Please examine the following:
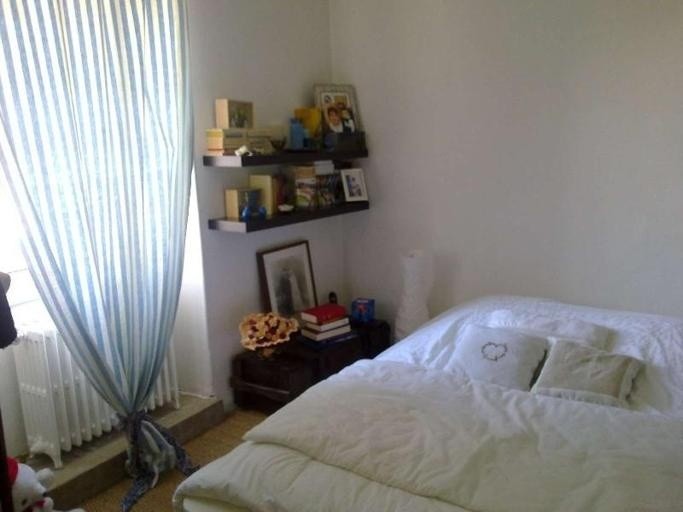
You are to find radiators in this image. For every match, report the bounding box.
[10,320,181,470]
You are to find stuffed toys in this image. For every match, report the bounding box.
[5,455,85,512]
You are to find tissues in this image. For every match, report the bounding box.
[352,297,375,322]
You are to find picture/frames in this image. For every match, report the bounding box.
[339,168,369,203]
[255,239,318,330]
[313,84,363,132]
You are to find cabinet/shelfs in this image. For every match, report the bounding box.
[229,315,391,412]
[202,147,369,233]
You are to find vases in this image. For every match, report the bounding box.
[247,349,280,367]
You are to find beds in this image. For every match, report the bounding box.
[171,290,683,512]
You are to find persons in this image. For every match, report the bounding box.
[349,176,362,197]
[277,266,291,315]
[323,102,343,133]
[340,109,355,136]
[288,269,305,313]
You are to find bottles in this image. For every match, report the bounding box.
[289,117,304,150]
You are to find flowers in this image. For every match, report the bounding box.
[238,311,300,352]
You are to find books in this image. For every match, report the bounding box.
[248,173,286,219]
[296,304,359,352]
[205,128,273,158]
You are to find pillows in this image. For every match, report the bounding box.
[534,337,642,408]
[441,323,548,391]
[490,308,612,349]
